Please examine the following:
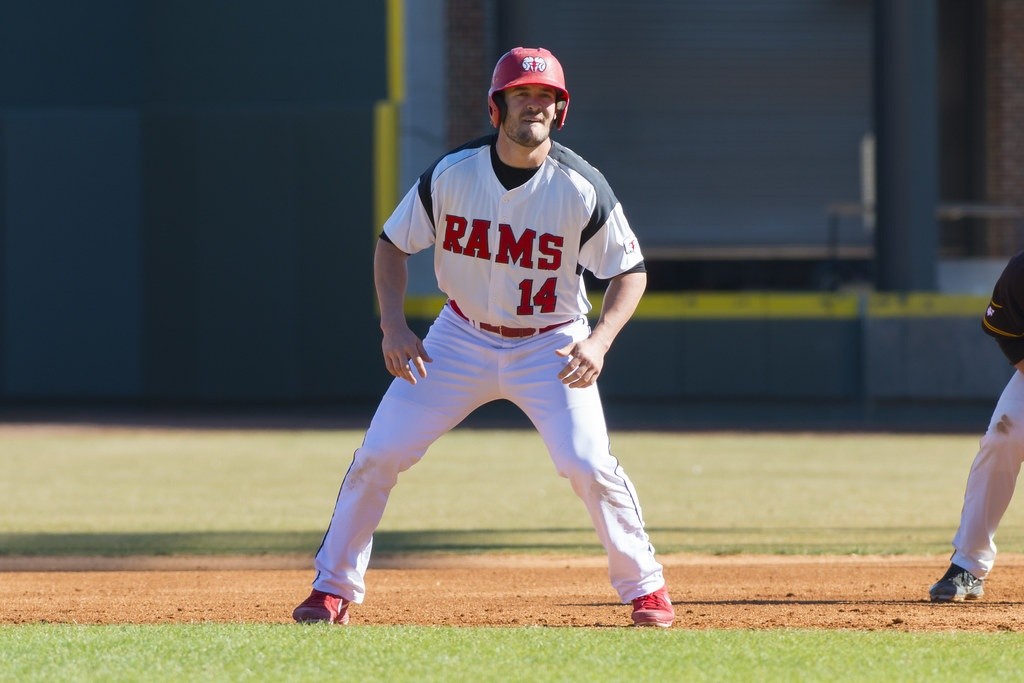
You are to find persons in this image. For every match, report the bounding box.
[929,252,1024,601]
[292,47,676,629]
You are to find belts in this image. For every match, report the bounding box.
[450,300,574,337]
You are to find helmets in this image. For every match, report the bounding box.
[487,46,570,131]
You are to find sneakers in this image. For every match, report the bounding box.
[631,584,675,627]
[293,587,349,625]
[929,563,985,603]
